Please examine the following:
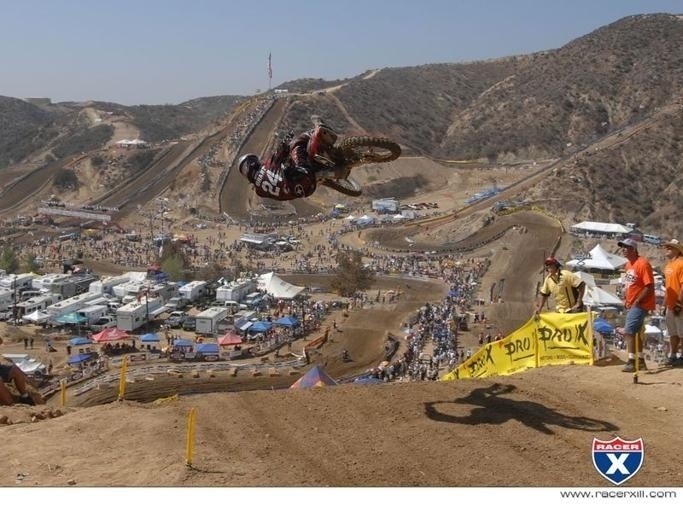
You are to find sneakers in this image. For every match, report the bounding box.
[311,115,324,126]
[21,396,36,406]
[662,356,683,367]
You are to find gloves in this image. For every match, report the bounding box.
[672,300,681,316]
[663,308,666,316]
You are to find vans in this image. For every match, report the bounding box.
[643,233,662,248]
[224,292,262,311]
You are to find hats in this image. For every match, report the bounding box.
[617,239,637,248]
[660,239,683,256]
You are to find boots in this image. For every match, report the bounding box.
[622,357,647,372]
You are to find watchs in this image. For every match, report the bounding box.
[635,299,640,304]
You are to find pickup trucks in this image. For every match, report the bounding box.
[90,316,117,332]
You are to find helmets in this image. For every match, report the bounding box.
[238,153,259,176]
[545,256,558,265]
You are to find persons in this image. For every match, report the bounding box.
[238,115,332,202]
[617,238,656,372]
[535,256,585,313]
[0,209,505,406]
[661,238,683,368]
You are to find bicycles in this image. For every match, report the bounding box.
[277,115,402,197]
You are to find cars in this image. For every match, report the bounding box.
[164,296,196,330]
[289,236,300,245]
[410,202,440,210]
